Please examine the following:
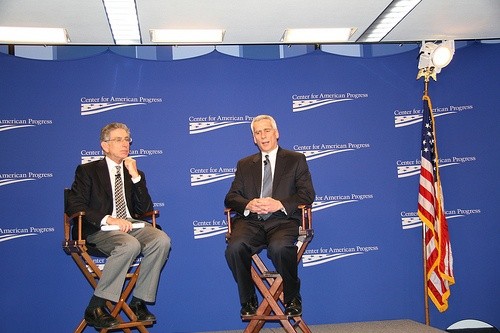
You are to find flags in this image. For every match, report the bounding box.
[417,95,455,312]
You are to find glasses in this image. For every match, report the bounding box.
[105,137,133,144]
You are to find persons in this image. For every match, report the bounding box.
[226,114,316,317]
[66,122,170,327]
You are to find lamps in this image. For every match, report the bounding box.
[419,42,455,71]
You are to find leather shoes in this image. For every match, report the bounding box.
[284,295,302,315]
[128,296,156,322]
[240,294,258,316]
[84,305,120,326]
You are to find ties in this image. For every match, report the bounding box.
[115,166,128,221]
[259,155,273,221]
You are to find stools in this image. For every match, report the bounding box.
[63,188,160,333]
[225,203,313,333]
